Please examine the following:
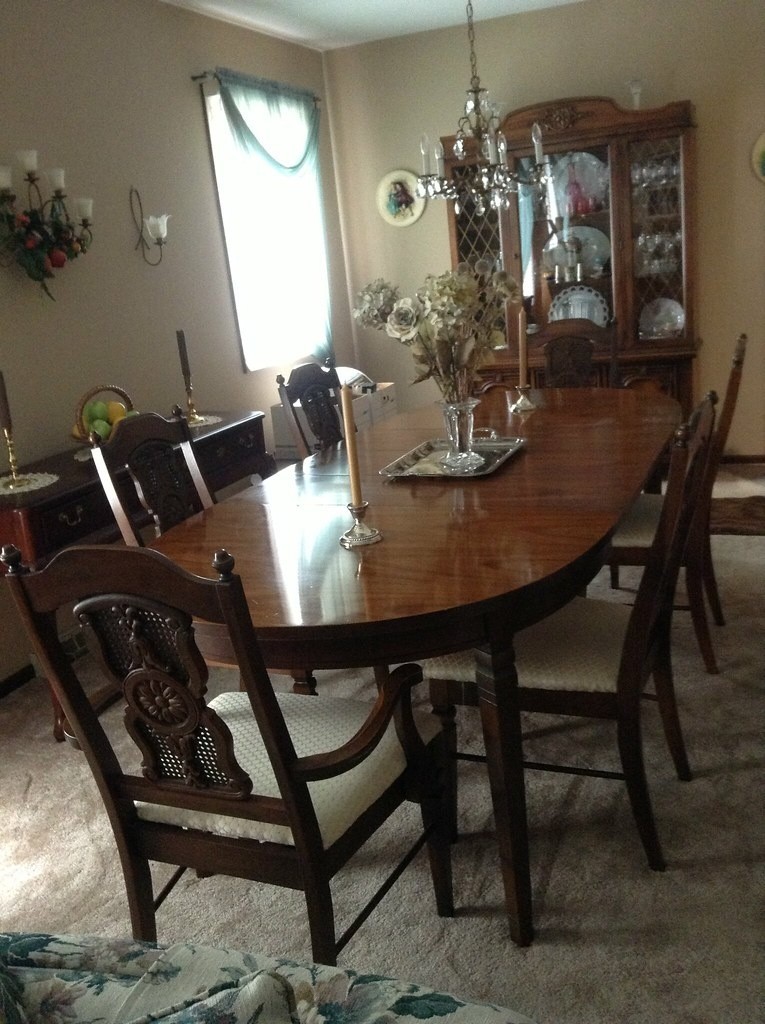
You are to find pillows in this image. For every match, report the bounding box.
[129,969,301,1024]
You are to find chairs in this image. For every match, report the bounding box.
[526,316,619,389]
[387,332,748,949]
[0,543,457,968]
[87,403,391,704]
[279,356,362,461]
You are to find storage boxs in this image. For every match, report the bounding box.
[271,381,397,460]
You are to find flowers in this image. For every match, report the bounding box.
[348,264,520,400]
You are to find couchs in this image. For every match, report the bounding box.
[0,931,538,1024]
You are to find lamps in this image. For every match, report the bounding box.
[0,146,93,269]
[415,0,551,218]
[130,184,172,268]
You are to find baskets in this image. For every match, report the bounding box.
[71,384,134,447]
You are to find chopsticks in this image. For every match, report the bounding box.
[540,226,611,279]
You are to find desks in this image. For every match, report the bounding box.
[0,408,276,742]
[105,384,682,942]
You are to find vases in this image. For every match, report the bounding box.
[437,395,486,473]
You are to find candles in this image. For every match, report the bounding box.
[0,370,12,428]
[340,380,363,507]
[176,330,190,377]
[518,306,527,388]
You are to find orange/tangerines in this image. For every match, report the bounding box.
[108,402,127,428]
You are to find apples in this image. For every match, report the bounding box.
[72,401,111,440]
[126,411,140,417]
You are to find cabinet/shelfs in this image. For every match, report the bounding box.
[438,93,704,483]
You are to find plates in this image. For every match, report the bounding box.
[548,285,610,322]
[638,298,684,340]
[546,152,610,216]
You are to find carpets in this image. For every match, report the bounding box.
[710,495,765,536]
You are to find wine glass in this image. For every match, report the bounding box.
[629,160,682,274]
